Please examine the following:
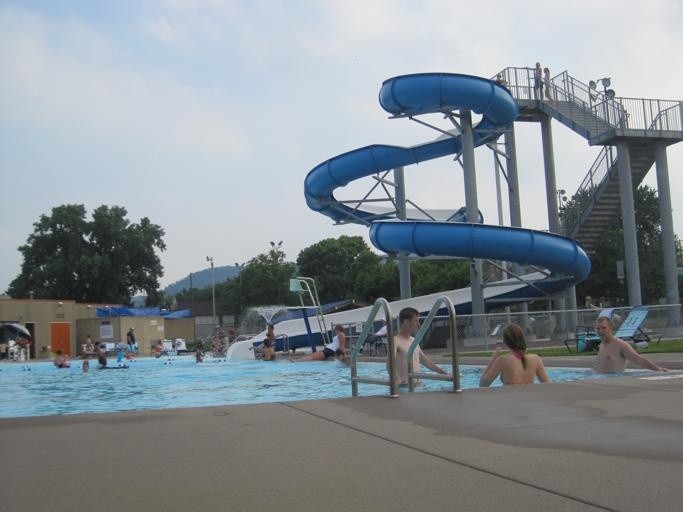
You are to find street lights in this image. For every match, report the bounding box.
[207,255,219,325]
[271,240,282,301]
[233,262,245,312]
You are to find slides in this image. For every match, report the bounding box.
[254,72,591,361]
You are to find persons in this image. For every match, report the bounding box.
[288,324,346,362]
[533,63,543,100]
[385,306,452,387]
[593,316,669,372]
[496,73,502,84]
[544,67,553,100]
[0,325,277,371]
[357,317,388,356]
[479,322,550,387]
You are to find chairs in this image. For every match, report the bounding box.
[362,319,387,357]
[564,306,650,355]
[162,336,191,356]
